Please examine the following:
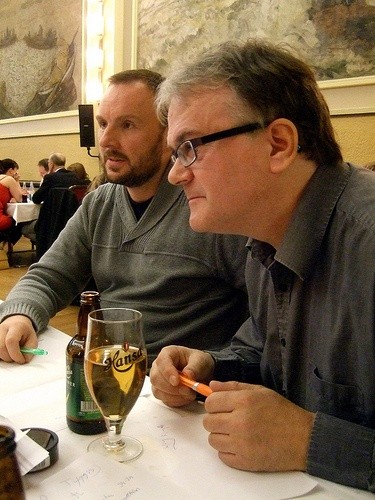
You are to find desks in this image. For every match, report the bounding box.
[5,197,44,266]
[0,299,375,500]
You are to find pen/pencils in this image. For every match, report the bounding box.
[179,374,213,398]
[20,347,48,355]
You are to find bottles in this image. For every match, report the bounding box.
[0,424,26,500]
[29,182,35,201]
[65,290,113,436]
[22,182,28,203]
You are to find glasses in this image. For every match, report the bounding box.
[170,119,303,167]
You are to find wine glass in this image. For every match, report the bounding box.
[83,308,148,463]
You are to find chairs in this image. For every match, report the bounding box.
[34,185,88,259]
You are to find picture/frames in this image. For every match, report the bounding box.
[0,0,86,125]
[131,0,375,89]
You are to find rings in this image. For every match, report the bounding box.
[16,172,17,174]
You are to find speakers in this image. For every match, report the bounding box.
[79,104,95,147]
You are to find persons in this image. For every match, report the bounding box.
[21,152,91,253]
[150,37,375,494]
[0,158,23,249]
[0,68,249,376]
